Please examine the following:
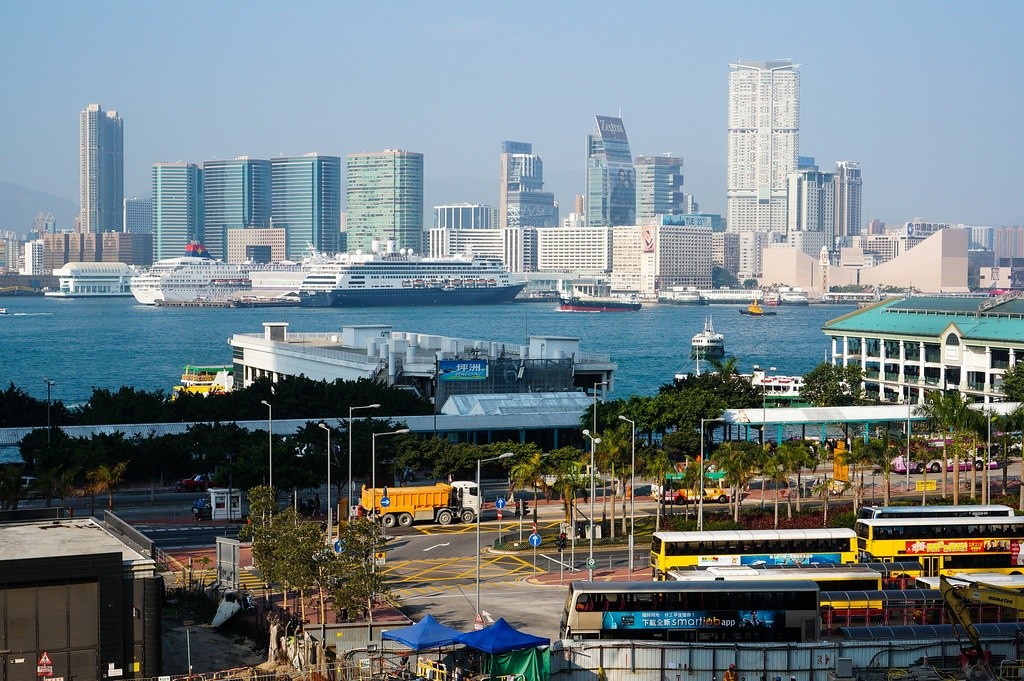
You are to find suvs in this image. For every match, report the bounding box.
[181,472,222,492]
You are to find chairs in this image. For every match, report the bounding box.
[582,594,691,611]
[873,527,1015,536]
[667,538,851,555]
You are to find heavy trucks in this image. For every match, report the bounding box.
[361,476,486,529]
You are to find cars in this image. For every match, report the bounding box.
[16,476,44,500]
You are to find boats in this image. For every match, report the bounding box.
[411,274,498,291]
[739,285,782,316]
[673,344,850,404]
[689,313,726,360]
[699,294,710,305]
[552,283,643,313]
[169,357,234,403]
[42,237,530,308]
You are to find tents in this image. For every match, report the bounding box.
[380,613,550,676]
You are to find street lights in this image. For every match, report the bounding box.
[318,423,332,591]
[476,451,515,623]
[594,381,607,501]
[372,427,411,603]
[619,415,635,570]
[348,403,381,537]
[698,417,726,532]
[42,376,55,450]
[581,428,601,582]
[260,399,272,531]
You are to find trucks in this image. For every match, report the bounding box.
[533,464,602,489]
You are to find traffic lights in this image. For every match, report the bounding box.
[514,501,530,517]
[555,533,567,551]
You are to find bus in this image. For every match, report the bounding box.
[650,472,744,504]
[557,503,1024,643]
[887,431,1024,475]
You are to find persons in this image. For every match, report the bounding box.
[674,463,686,473]
[174,480,182,493]
[765,440,774,453]
[608,168,636,227]
[749,611,761,626]
[984,540,1010,552]
[358,494,362,505]
[643,229,654,252]
[312,493,324,517]
[817,433,852,461]
[790,435,801,441]
[722,663,739,681]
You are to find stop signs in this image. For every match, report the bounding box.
[532,522,537,534]
[497,510,503,521]
